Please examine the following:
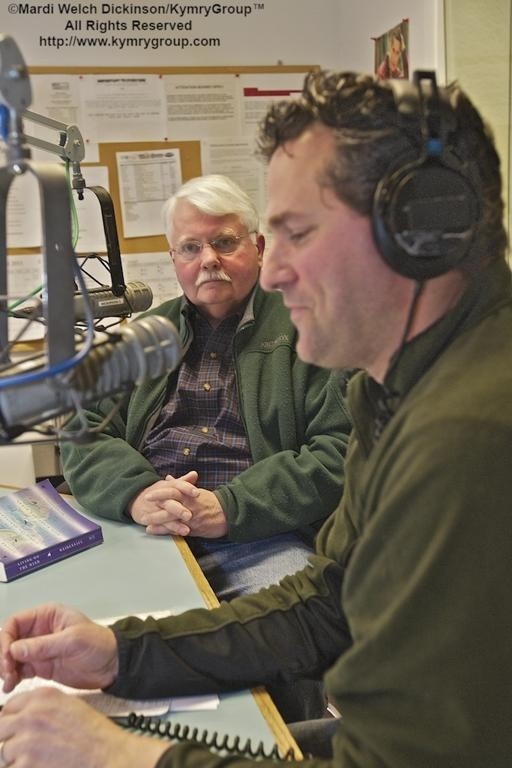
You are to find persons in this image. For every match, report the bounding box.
[59,175,352,600]
[0,65,511,768]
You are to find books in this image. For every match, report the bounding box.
[0,478,103,582]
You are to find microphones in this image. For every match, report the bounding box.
[9,283,152,317]
[2,316,183,442]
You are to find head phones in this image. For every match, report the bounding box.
[369,67,480,285]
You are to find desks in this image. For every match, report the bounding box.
[0,456,316,766]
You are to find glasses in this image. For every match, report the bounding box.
[169,230,259,260]
